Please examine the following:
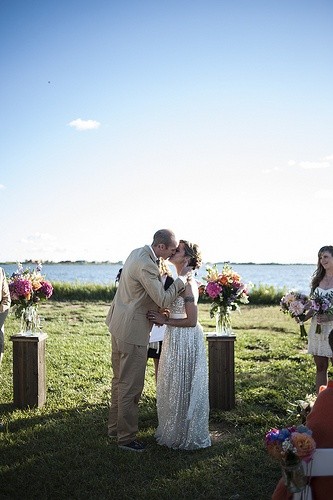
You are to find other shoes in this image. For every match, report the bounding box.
[121,438,148,453]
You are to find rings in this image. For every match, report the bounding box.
[153,317,155,319]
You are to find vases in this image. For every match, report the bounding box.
[216,308,232,336]
[283,464,307,493]
[20,305,40,335]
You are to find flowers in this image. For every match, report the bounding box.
[6,260,54,305]
[263,424,317,463]
[199,261,249,318]
[280,288,333,338]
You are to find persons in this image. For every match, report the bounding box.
[146,240,211,450]
[147,260,174,384]
[295,245,333,394]
[104,230,192,452]
[0,267,10,366]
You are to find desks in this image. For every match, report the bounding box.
[10,333,49,408]
[206,334,237,413]
